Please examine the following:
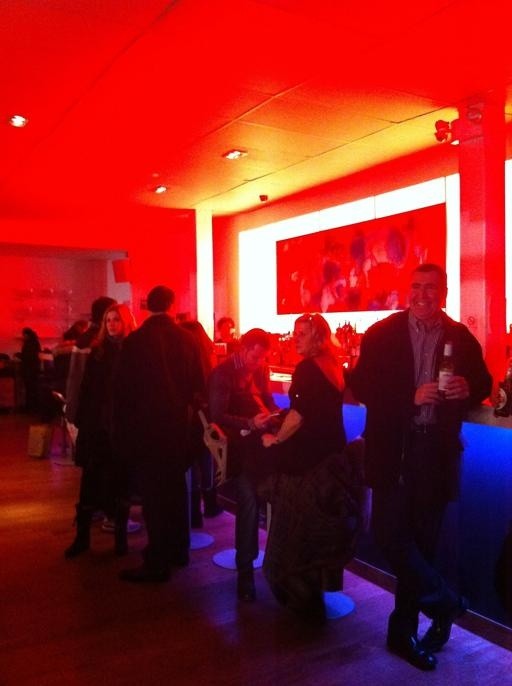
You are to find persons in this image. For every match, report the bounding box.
[18,319,84,425]
[348,265,491,670]
[205,313,346,641]
[318,230,408,310]
[64,284,238,581]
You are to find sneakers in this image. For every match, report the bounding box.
[235,556,256,593]
[120,566,170,582]
[115,532,127,555]
[101,518,141,533]
[141,546,187,567]
[64,538,89,556]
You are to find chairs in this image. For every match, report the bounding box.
[50,389,67,407]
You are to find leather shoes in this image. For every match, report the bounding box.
[386,629,437,669]
[421,597,468,651]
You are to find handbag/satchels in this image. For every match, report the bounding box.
[195,421,229,492]
[28,427,51,458]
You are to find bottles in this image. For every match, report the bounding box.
[494,363,512,418]
[437,339,455,398]
